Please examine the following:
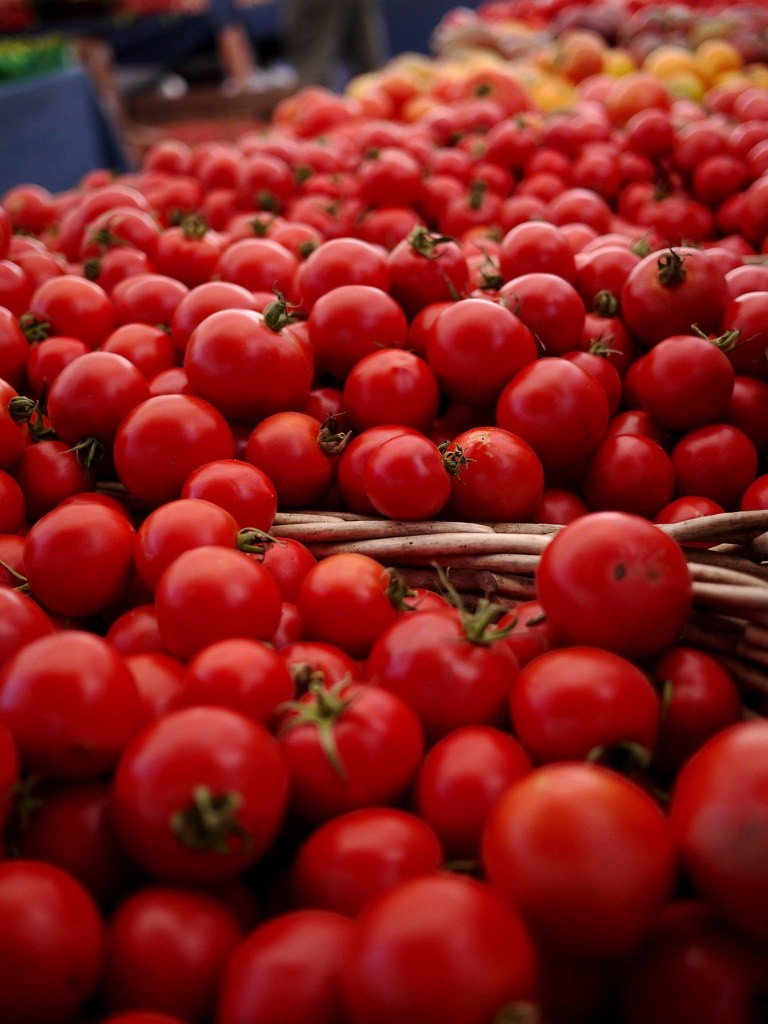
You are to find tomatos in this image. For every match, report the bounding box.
[0,0,768,1024]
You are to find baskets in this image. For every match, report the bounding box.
[25,469,768,532]
[0,534,768,721]
